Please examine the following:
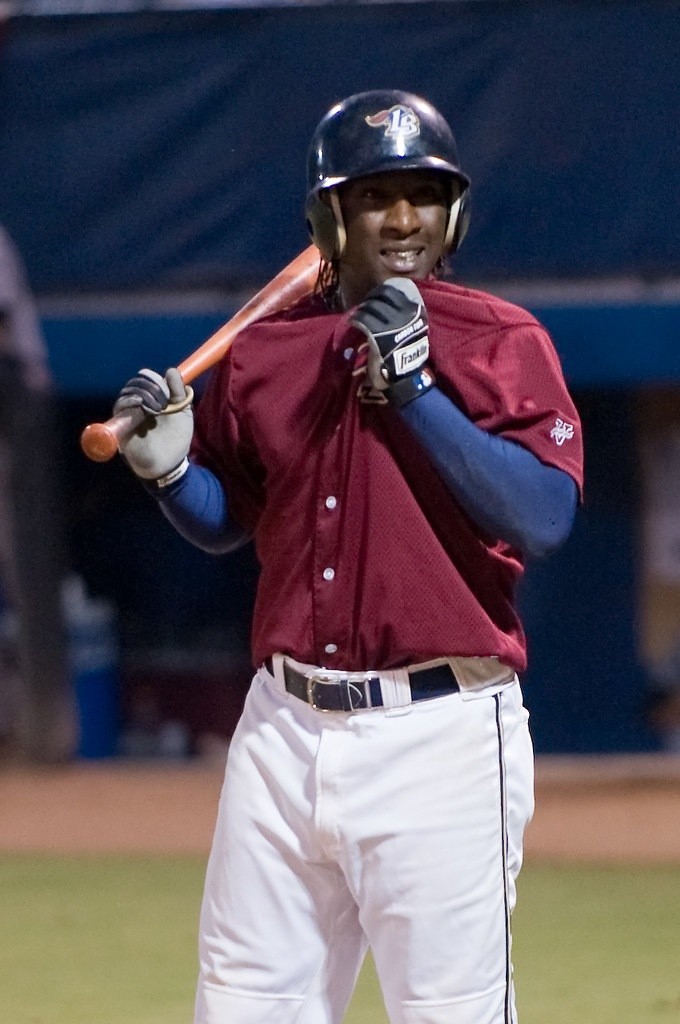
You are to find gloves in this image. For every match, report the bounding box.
[114,367,196,491]
[348,276,438,412]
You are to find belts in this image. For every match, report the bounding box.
[263,657,460,713]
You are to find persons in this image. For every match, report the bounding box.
[0,224,83,763]
[114,90,581,1023]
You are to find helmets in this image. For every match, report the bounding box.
[300,88,472,263]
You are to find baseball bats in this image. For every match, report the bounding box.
[78,243,338,463]
